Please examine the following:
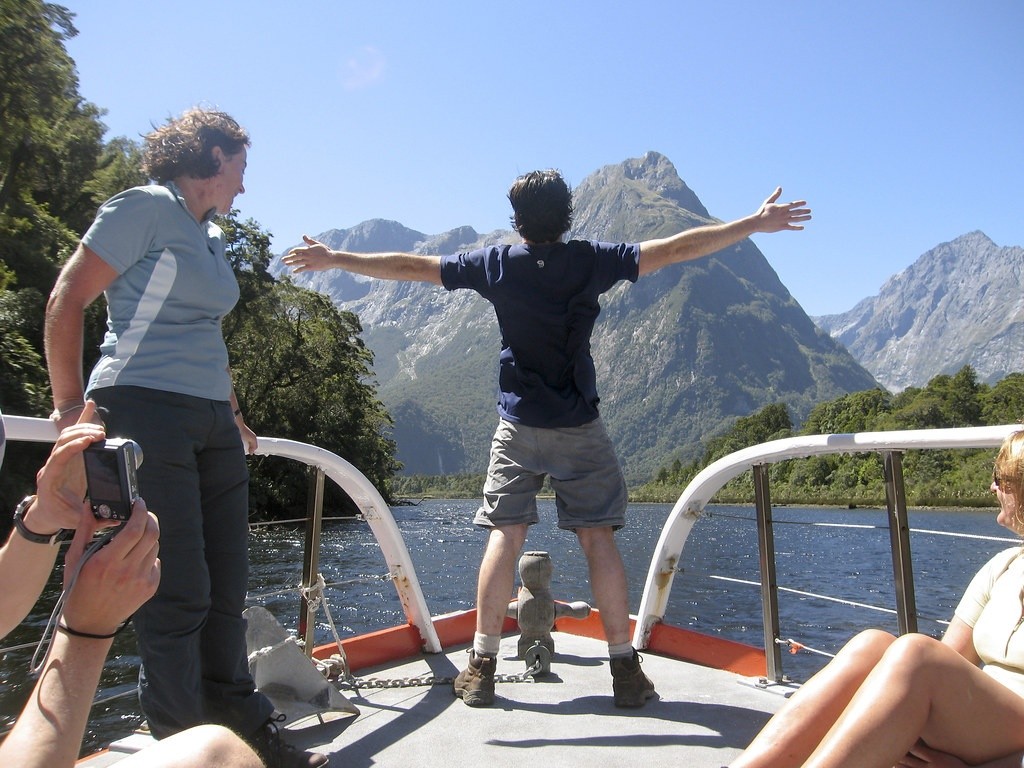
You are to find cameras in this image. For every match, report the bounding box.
[83,439,145,522]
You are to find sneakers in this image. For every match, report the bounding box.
[610,646,654,707]
[247,714,330,768]
[453,646,497,707]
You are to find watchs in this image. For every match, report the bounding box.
[11,495,65,543]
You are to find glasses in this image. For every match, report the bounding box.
[994,472,999,486]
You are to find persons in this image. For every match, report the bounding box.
[283,171,811,705]
[46,110,329,768]
[0,399,266,768]
[727,431,1024,768]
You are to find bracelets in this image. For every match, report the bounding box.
[56,616,130,640]
[232,409,240,418]
